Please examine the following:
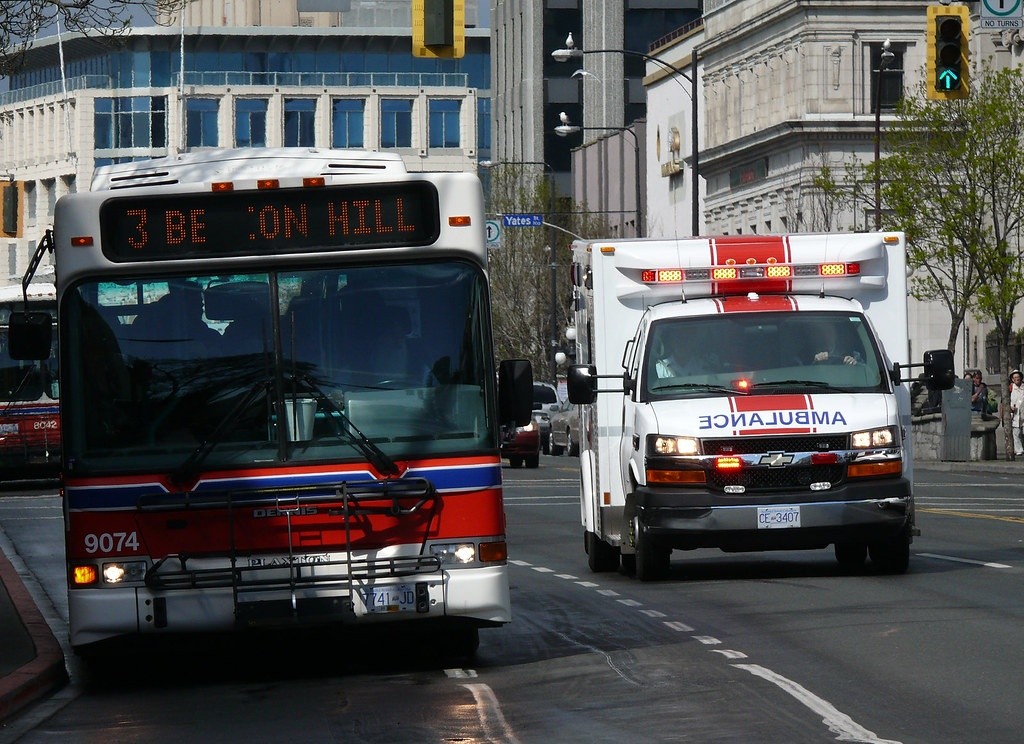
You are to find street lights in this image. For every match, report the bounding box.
[548,32,703,238]
[873,39,895,234]
[552,110,640,241]
[477,157,560,389]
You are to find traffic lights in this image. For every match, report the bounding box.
[926,4,973,101]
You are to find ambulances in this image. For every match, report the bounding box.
[567,231,957,583]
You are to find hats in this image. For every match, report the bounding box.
[1010,370,1023,382]
[973,372,982,376]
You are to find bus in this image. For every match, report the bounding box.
[6,142,514,661]
[0,283,63,477]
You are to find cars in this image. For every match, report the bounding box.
[532,381,560,431]
[502,415,542,470]
[544,396,584,457]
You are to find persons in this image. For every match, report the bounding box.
[85,280,284,436]
[963,371,988,414]
[348,306,437,390]
[796,324,866,365]
[910,373,942,415]
[1009,369,1024,456]
